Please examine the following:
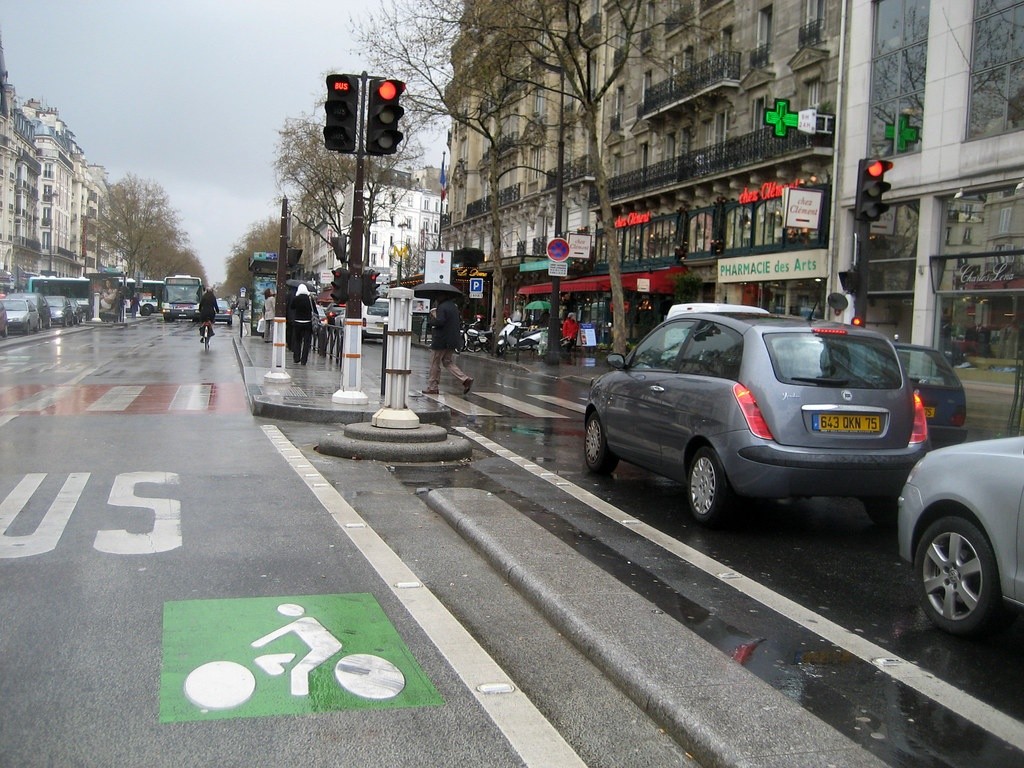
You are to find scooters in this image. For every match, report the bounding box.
[454,310,548,359]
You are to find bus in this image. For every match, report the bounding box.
[29,274,206,323]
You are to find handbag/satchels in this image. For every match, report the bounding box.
[257,318,265,333]
[311,312,320,334]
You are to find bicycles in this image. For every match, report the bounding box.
[201,312,219,354]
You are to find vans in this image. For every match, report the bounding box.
[334,299,389,343]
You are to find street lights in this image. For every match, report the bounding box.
[44,193,59,276]
[466,26,566,365]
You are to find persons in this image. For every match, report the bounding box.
[130,293,139,318]
[964,319,1017,360]
[563,313,579,356]
[285,289,297,352]
[263,288,275,342]
[422,294,474,396]
[539,309,550,328]
[198,288,220,342]
[100,280,118,311]
[119,296,125,322]
[290,284,320,366]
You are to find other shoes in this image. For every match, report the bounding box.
[211,333,215,336]
[463,377,475,394]
[422,387,440,394]
[200,336,204,343]
[294,360,300,363]
[301,361,306,365]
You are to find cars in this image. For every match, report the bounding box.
[891,342,968,451]
[584,303,930,536]
[316,303,346,326]
[0,292,83,338]
[214,298,251,326]
[896,435,1024,642]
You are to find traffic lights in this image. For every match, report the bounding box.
[851,317,862,326]
[330,234,346,262]
[839,272,860,293]
[368,79,406,154]
[323,74,358,153]
[855,158,894,223]
[331,268,348,304]
[363,269,381,306]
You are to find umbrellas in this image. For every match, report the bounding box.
[412,281,468,302]
[525,301,550,310]
[286,280,317,292]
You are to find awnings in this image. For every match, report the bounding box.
[518,266,684,295]
[520,257,574,274]
